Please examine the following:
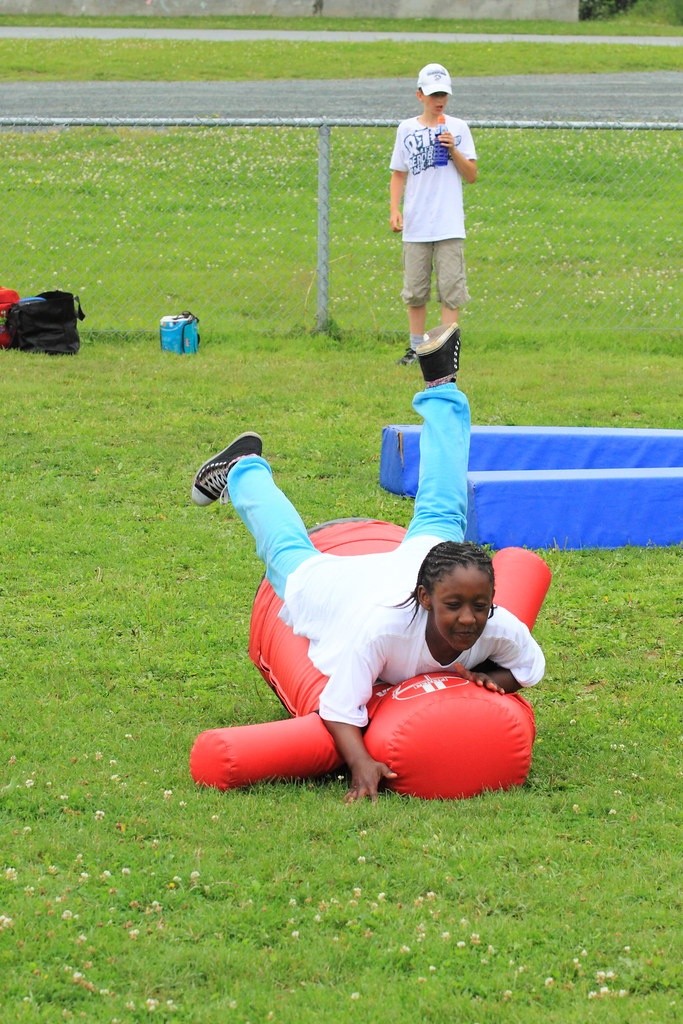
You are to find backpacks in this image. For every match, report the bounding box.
[162,310,198,354]
[0,287,81,355]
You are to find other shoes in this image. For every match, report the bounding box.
[398,350,419,367]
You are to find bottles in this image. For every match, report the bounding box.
[434,115,449,166]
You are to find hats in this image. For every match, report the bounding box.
[419,63,454,96]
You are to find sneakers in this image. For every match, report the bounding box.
[194,432,262,508]
[417,324,463,382]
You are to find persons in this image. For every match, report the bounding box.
[390,63,478,369]
[191,321,548,808]
